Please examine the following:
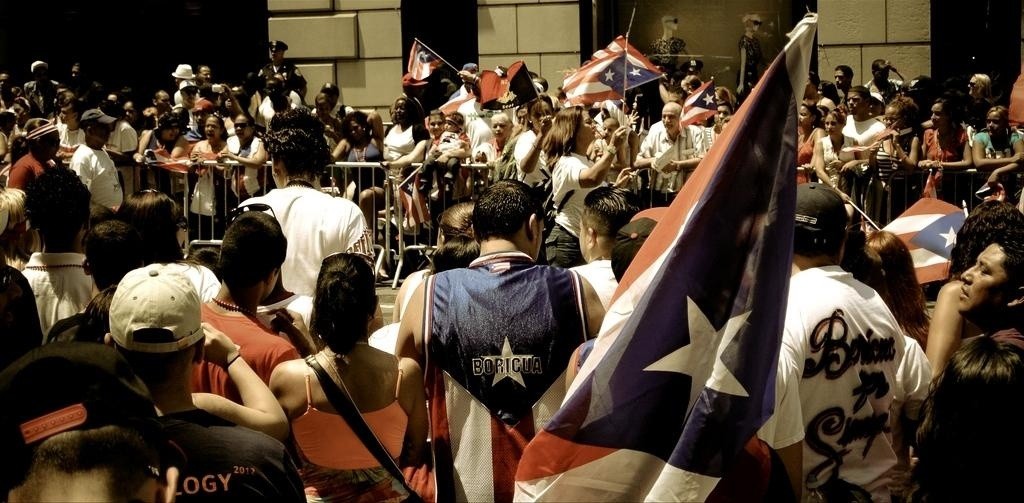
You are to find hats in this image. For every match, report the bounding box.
[1,341,189,468]
[108,264,205,356]
[611,206,670,282]
[171,63,196,79]
[179,80,200,91]
[795,181,854,233]
[152,111,185,132]
[319,82,339,96]
[77,109,119,129]
[267,41,288,52]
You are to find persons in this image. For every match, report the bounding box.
[0,1,1019,501]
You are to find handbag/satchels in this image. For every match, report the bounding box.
[190,171,217,216]
